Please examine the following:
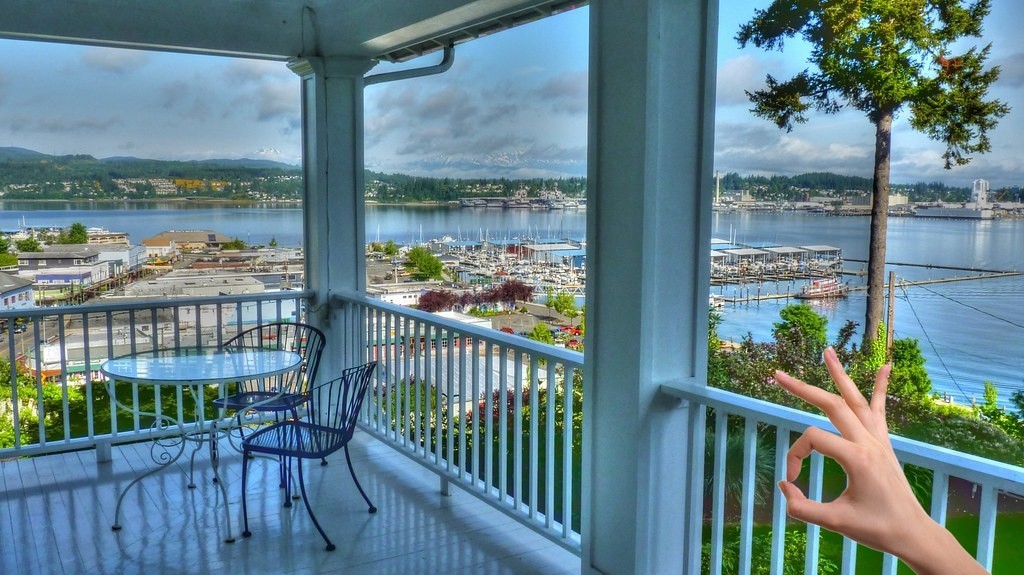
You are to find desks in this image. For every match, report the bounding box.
[100,346,303,545]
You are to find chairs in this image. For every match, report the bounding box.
[214,322,328,488]
[240,361,378,551]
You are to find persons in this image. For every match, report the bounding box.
[776,346,991,575]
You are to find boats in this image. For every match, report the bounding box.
[459,198,487,207]
[548,202,563,210]
[794,275,849,299]
[565,201,579,208]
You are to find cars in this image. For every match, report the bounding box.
[499,325,582,350]
[262,334,277,340]
[13,324,27,334]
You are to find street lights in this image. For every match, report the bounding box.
[39,285,48,307]
